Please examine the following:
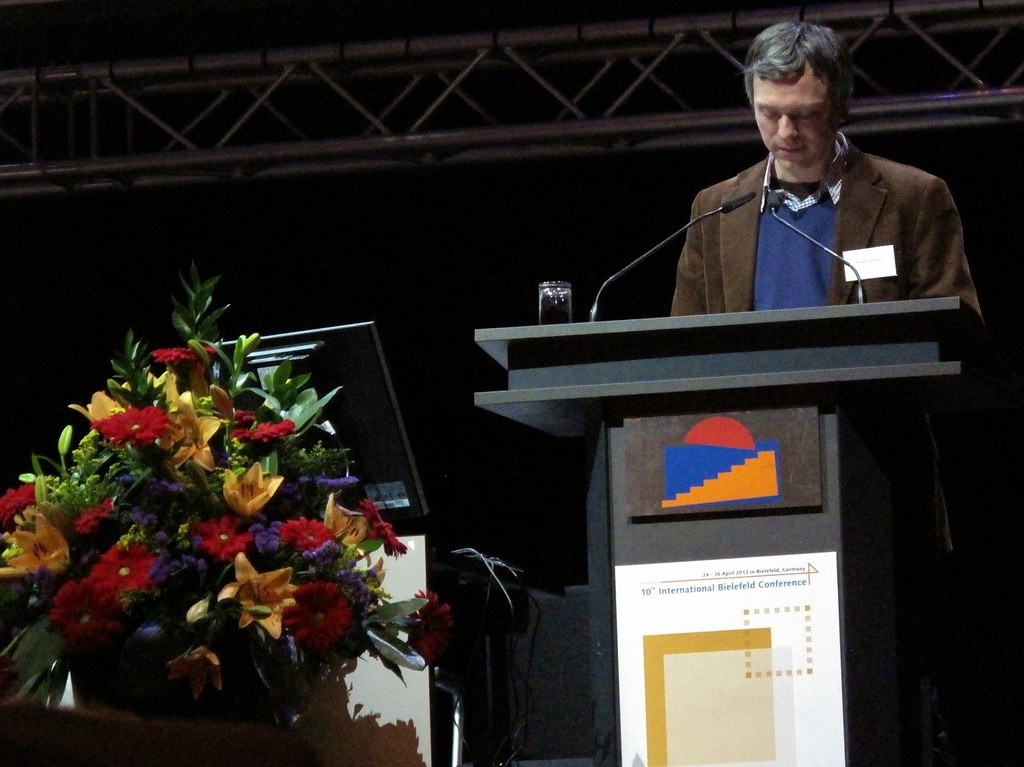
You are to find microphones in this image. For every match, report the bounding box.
[767,191,867,306]
[590,190,759,321]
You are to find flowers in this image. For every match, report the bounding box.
[2,269,460,726]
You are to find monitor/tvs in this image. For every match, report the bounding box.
[207,321,428,526]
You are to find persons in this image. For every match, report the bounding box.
[670,21,981,766]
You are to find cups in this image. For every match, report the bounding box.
[537,280,574,325]
